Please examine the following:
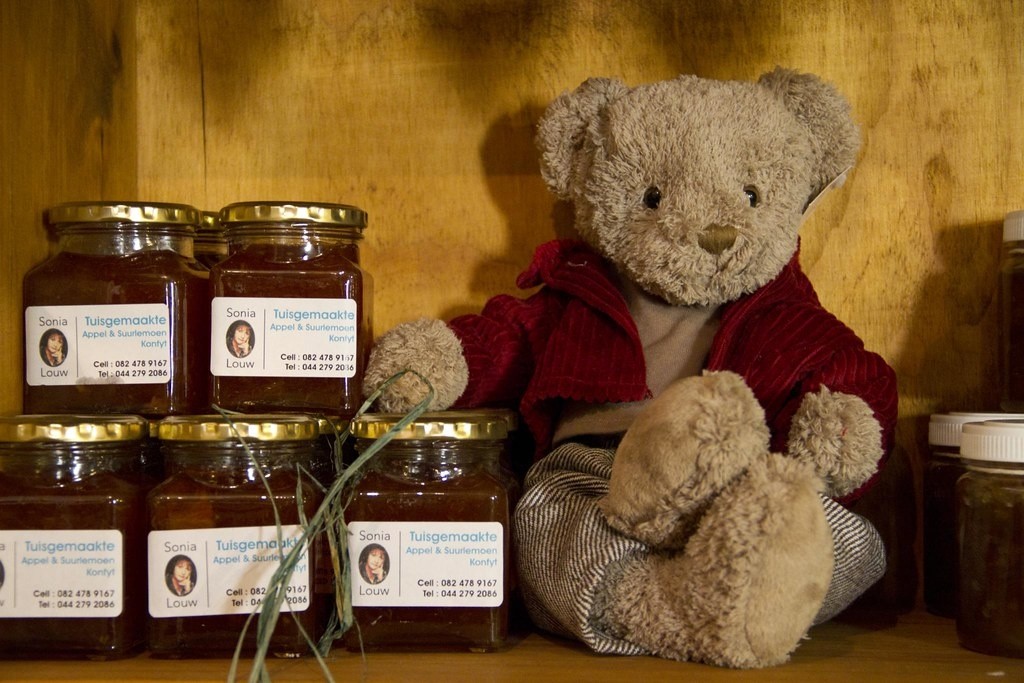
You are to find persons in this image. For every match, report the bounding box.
[166,555,196,596]
[39,328,68,367]
[359,544,390,584]
[225,320,255,358]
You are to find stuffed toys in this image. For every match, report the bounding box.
[360,66,899,671]
